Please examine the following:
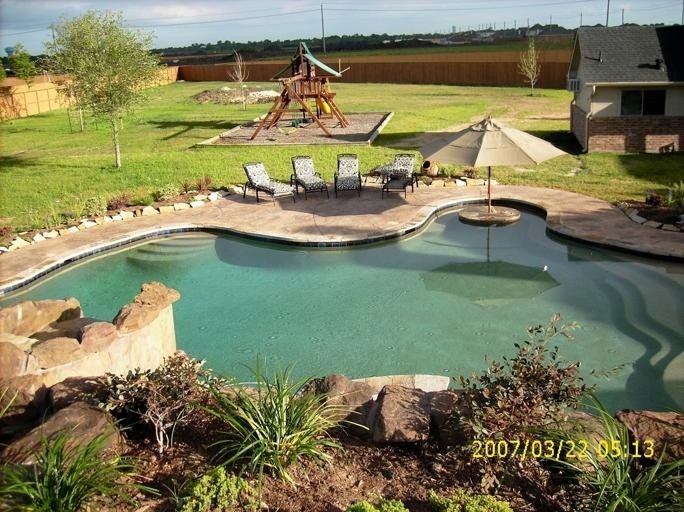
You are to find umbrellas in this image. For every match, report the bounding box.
[417,225,562,306]
[416,115,568,213]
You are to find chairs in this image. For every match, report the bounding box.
[362,166,419,187]
[242,161,295,207]
[290,155,329,200]
[382,154,413,200]
[334,153,361,199]
